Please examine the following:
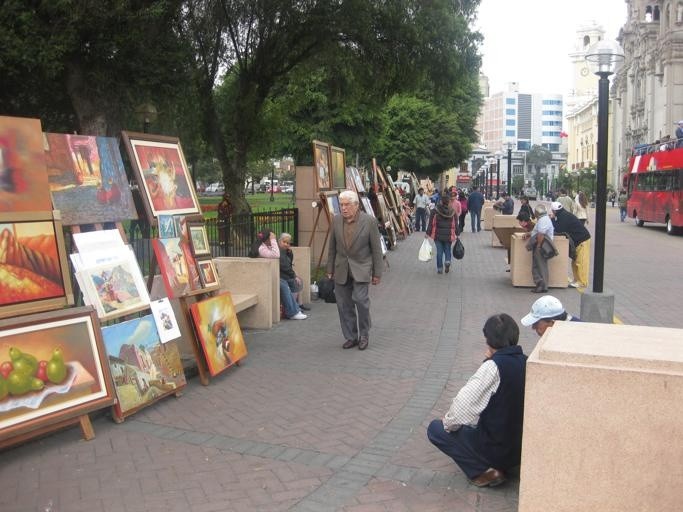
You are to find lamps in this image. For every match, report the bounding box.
[309,198,321,216]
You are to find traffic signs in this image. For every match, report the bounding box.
[470,137,522,200]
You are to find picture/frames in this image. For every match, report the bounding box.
[327,146,347,191]
[317,193,342,221]
[186,220,210,253]
[194,259,222,288]
[123,130,204,223]
[1,211,75,318]
[312,140,332,192]
[351,166,415,252]
[0,305,121,451]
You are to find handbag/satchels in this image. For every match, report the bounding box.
[318,277,337,303]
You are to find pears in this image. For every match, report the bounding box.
[0,344,67,400]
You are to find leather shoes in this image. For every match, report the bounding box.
[343,340,358,349]
[465,468,505,488]
[359,335,369,350]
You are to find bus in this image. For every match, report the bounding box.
[620,135,683,237]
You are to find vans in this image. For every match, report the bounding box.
[526,187,537,199]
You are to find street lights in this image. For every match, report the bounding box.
[539,169,596,201]
[583,25,629,294]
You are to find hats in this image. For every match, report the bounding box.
[521,295,565,327]
[550,202,563,211]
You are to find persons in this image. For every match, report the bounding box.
[402,184,495,233]
[247,228,307,320]
[424,194,460,274]
[606,187,631,222]
[324,191,383,351]
[674,120,683,148]
[276,232,311,313]
[426,313,528,488]
[495,186,596,293]
[630,135,676,156]
[519,294,581,338]
[216,192,234,249]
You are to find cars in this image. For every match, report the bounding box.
[192,177,294,193]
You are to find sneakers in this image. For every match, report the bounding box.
[531,288,547,293]
[568,280,585,287]
[299,304,311,310]
[290,311,307,320]
[445,263,450,273]
[438,270,443,274]
